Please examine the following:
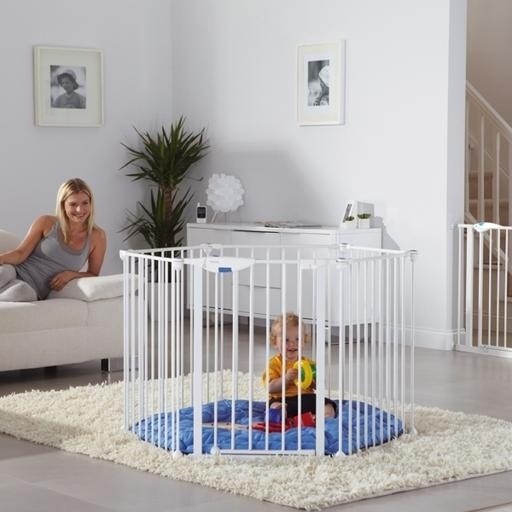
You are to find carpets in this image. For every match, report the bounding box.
[0,367,512,511]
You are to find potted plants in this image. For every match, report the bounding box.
[116,112,211,325]
[342,212,372,229]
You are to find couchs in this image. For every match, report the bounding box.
[0,228,150,376]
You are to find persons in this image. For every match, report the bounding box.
[313,66,329,104]
[51,69,85,108]
[261,312,337,421]
[0,177,106,301]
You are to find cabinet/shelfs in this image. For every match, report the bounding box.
[184,222,385,347]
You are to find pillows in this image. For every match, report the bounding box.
[46,274,146,306]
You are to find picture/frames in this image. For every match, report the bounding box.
[29,44,105,129]
[295,38,348,127]
